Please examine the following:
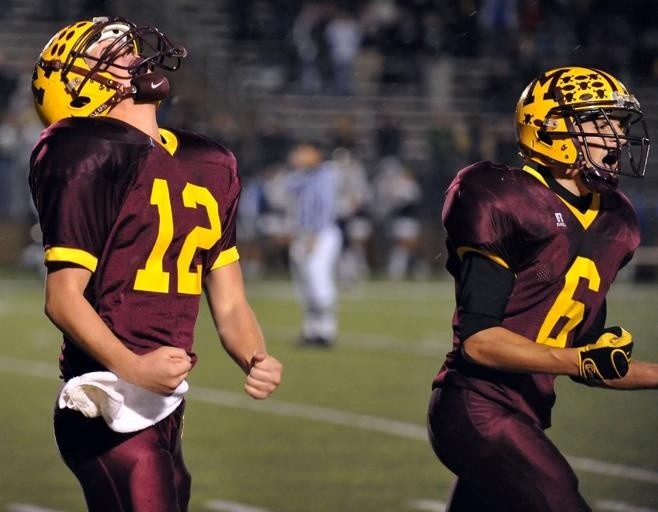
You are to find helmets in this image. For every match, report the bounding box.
[31,18,141,133]
[513,64,644,173]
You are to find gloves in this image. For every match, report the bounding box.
[575,324,635,384]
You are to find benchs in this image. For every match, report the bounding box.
[1,0,515,169]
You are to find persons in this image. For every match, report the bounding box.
[427,66,658,510]
[1,5,658,346]
[29,12,284,511]
